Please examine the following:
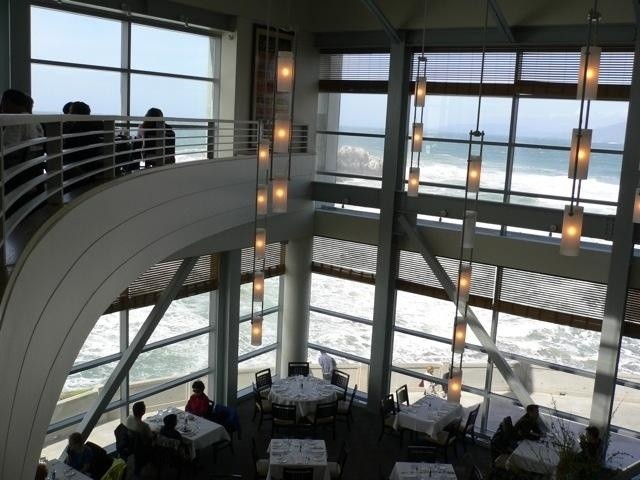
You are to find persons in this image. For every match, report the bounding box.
[185,381,209,417]
[123,402,154,438]
[319,351,335,380]
[66,433,92,476]
[441,365,452,398]
[515,405,545,441]
[423,366,436,396]
[577,427,603,464]
[1,89,175,167]
[35,464,48,480]
[491,416,518,454]
[159,414,183,442]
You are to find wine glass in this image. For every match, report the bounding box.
[155,405,200,436]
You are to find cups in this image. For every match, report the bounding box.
[41,457,75,480]
[407,395,457,480]
[271,373,331,464]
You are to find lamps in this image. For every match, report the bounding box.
[560,0,603,257]
[407,0,429,197]
[251,122,271,347]
[271,50,296,215]
[448,0,489,402]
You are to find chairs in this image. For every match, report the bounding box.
[255,368,272,390]
[186,399,214,420]
[269,403,297,439]
[288,362,309,376]
[396,384,410,413]
[302,402,336,441]
[379,394,413,449]
[574,439,605,477]
[490,431,520,478]
[454,403,481,448]
[212,407,242,458]
[333,385,357,431]
[408,446,437,463]
[115,424,157,476]
[433,417,468,462]
[502,416,519,451]
[454,453,483,479]
[331,369,349,401]
[252,383,273,432]
[327,441,348,478]
[152,435,195,476]
